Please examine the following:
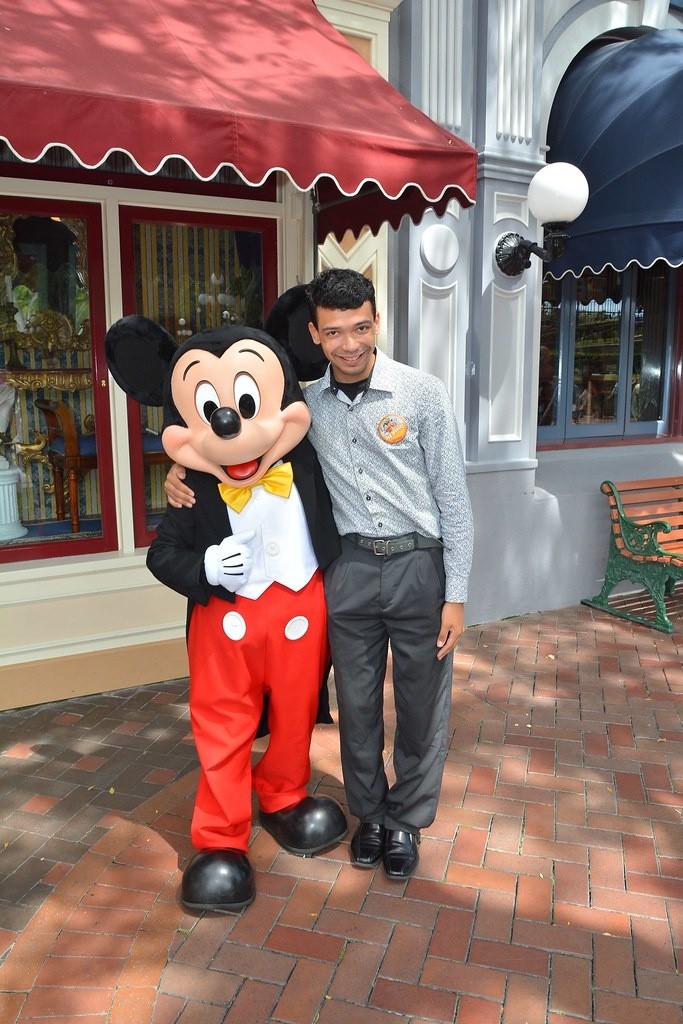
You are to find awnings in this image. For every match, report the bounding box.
[0,0,478,246]
[541,29,683,282]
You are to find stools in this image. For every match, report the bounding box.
[34,397,175,535]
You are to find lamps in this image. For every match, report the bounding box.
[493,161,589,279]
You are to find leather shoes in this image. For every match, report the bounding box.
[381,829,421,880]
[350,823,387,868]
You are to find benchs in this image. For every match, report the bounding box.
[579,474,683,634]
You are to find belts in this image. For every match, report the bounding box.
[346,532,452,556]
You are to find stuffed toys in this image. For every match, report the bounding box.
[104,283,348,911]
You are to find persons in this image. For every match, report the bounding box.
[163,266,475,880]
